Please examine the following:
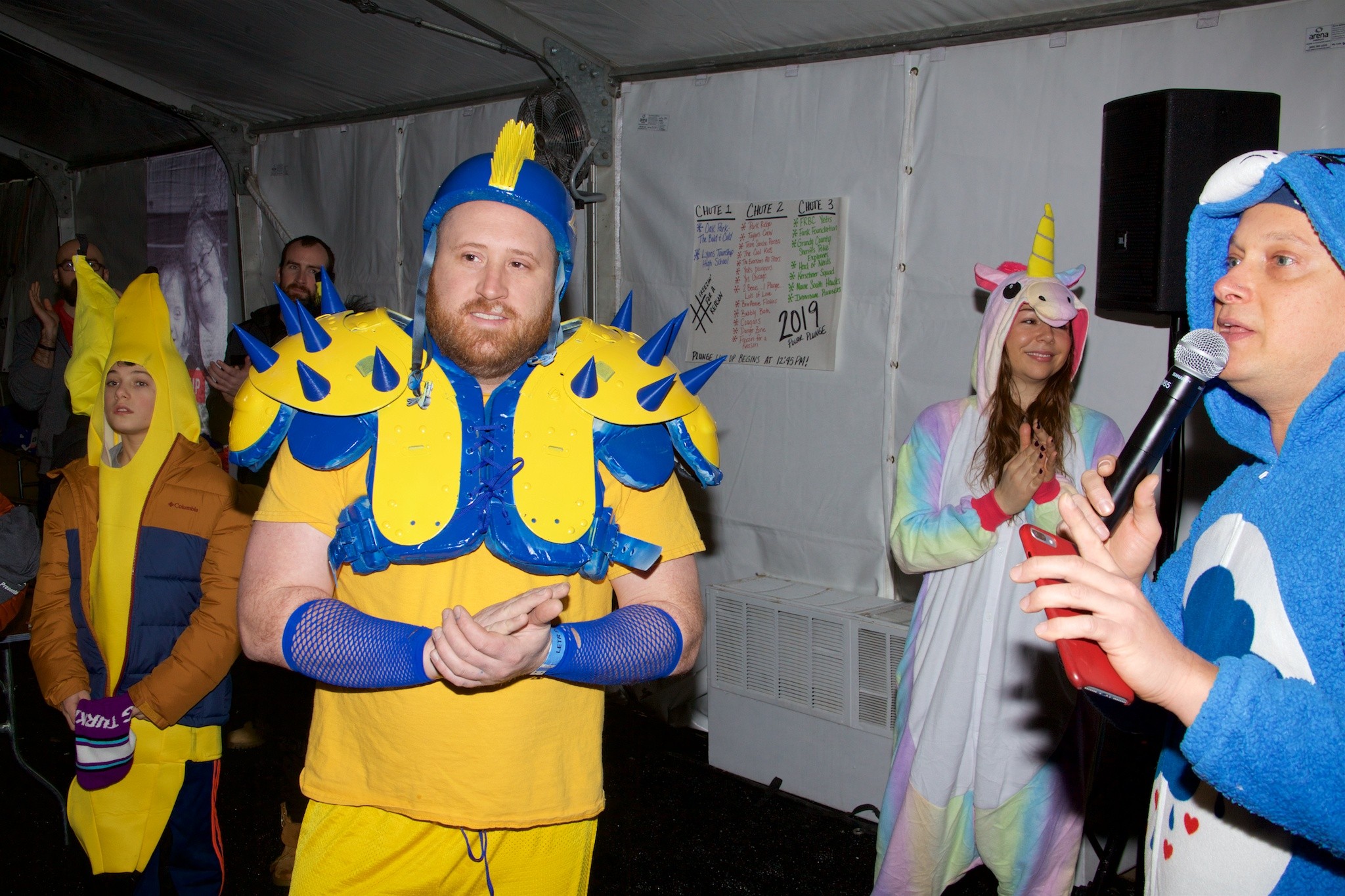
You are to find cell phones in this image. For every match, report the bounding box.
[1019,524,1135,705]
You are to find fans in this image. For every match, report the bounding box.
[517,85,606,204]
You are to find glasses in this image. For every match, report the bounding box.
[187,242,215,284]
[57,258,106,271]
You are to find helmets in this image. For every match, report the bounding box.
[407,118,577,302]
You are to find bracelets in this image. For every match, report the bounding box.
[39,344,56,352]
[530,626,566,675]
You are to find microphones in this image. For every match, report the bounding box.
[1092,328,1231,543]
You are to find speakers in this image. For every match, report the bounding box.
[1099,87,1283,329]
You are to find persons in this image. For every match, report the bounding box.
[230,118,724,895]
[1010,146,1345,896]
[206,235,336,421]
[8,240,123,526]
[0,492,42,631]
[158,213,240,444]
[872,203,1126,896]
[29,272,250,895]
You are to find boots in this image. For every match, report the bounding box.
[274,803,304,885]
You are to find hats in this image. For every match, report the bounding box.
[74,694,136,791]
[1261,181,1306,211]
